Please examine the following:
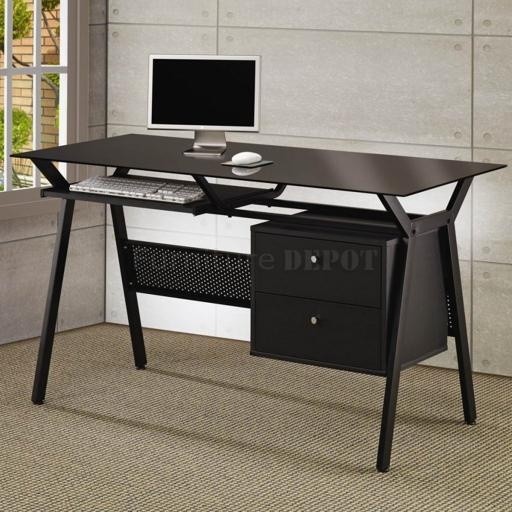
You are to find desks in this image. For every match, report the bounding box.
[10,132,508,472]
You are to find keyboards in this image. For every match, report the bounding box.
[69,175,204,204]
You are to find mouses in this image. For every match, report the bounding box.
[232,151,262,166]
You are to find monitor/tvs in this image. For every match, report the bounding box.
[146,54,260,158]
